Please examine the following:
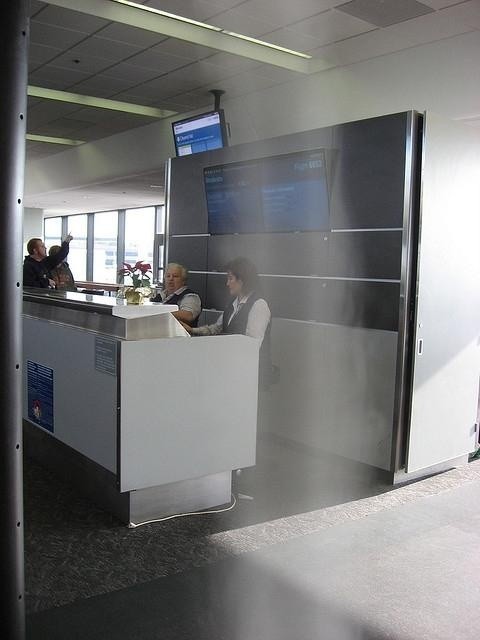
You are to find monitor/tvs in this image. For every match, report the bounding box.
[171,109,229,156]
[202,145,329,234]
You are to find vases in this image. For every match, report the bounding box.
[127,288,145,305]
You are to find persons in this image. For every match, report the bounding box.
[148,262,202,326]
[23,230,75,289]
[176,256,272,350]
[47,244,77,289]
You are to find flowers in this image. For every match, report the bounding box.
[115,260,155,293]
